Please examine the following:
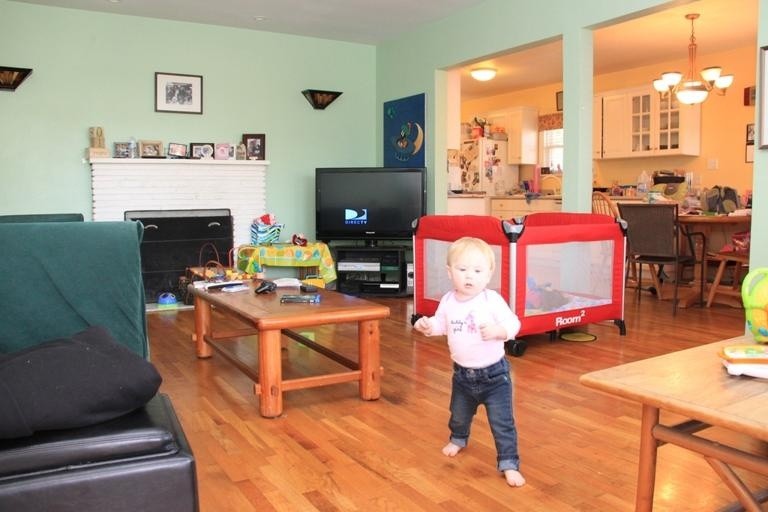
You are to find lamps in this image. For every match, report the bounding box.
[301,89,343,110]
[652,13,734,106]
[0,66,32,92]
[470,67,497,81]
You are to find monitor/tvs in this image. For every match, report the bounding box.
[316,166,427,244]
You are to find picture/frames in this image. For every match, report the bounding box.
[139,139,164,158]
[167,142,187,156]
[556,92,563,111]
[155,72,203,114]
[228,144,237,160]
[242,134,265,160]
[109,137,136,159]
[745,144,754,163]
[746,123,755,143]
[190,143,215,159]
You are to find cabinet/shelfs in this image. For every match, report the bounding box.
[489,194,643,221]
[487,106,538,165]
[329,245,411,294]
[593,88,629,159]
[630,85,701,158]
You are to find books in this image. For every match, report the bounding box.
[280,293,320,303]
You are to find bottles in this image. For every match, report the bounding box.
[129,136,138,159]
[626,185,635,197]
[612,184,620,196]
[663,185,689,215]
[490,127,507,140]
[648,191,661,204]
[637,171,650,197]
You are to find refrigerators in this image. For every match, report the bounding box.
[463,138,520,216]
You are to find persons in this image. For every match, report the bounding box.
[414,236,526,487]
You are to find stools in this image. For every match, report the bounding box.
[0,393,199,511]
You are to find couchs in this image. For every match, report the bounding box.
[0,215,147,358]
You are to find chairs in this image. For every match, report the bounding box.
[592,191,662,307]
[616,202,706,316]
[706,252,749,308]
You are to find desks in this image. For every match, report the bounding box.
[238,242,337,289]
[580,333,768,511]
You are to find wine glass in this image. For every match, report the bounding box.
[523,180,533,193]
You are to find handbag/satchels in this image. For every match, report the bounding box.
[701,184,740,215]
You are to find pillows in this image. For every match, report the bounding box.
[1,326,162,439]
